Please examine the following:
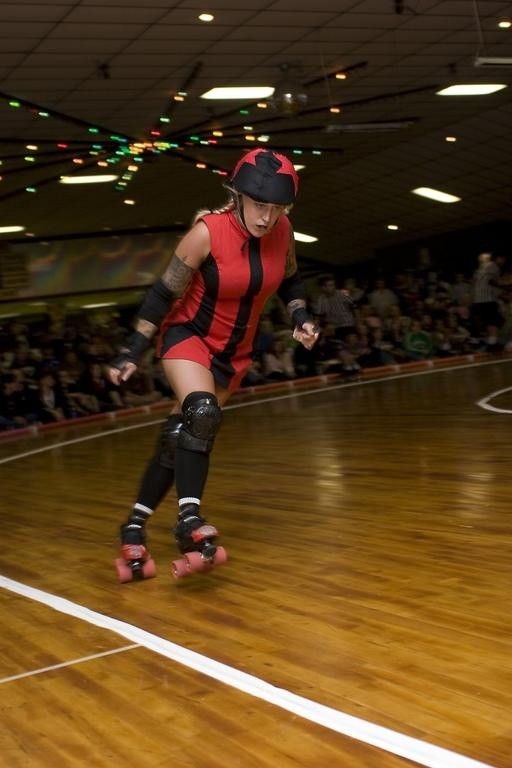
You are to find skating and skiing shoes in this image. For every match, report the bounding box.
[113,512,158,583]
[170,507,227,579]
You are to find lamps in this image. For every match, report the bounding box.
[318,27,420,135]
[473,0,512,69]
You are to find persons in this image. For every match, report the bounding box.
[102,148,322,582]
[1,300,178,432]
[240,246,512,388]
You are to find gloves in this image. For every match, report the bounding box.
[290,306,322,338]
[106,329,151,373]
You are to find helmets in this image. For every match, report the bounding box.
[224,148,298,238]
